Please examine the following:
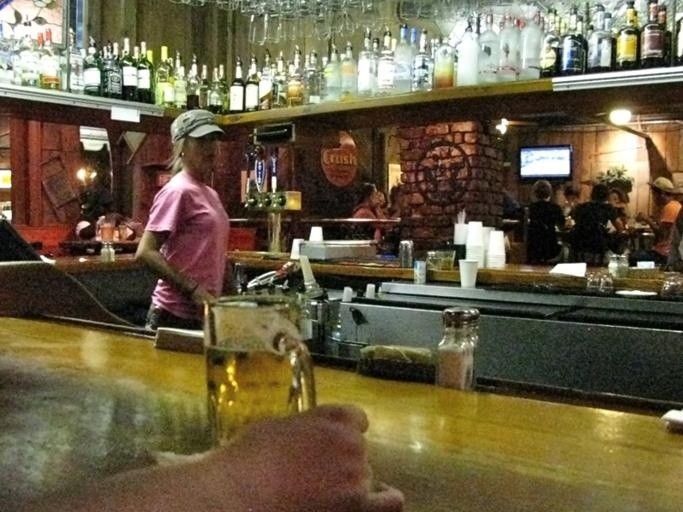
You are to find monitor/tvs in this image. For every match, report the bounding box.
[518,144,573,185]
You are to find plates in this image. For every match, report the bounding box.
[615,289,658,299]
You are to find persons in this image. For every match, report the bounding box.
[134,109,232,332]
[340,182,404,257]
[499,176,683,273]
[0,401,406,512]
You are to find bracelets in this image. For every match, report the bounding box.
[182,283,202,298]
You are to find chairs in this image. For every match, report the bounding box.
[569,223,609,266]
[515,223,558,265]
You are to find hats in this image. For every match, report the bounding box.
[646,177,673,194]
[170,108,226,140]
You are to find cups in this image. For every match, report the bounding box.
[201,295,315,450]
[428,249,456,270]
[660,272,683,298]
[587,272,614,290]
[398,239,416,268]
[171,0,513,45]
[463,219,506,270]
[289,238,305,261]
[307,225,324,242]
[458,257,478,289]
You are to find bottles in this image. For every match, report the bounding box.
[100,214,127,243]
[331,312,347,340]
[435,306,479,391]
[457,0,682,87]
[11,23,452,114]
[607,253,628,277]
[99,240,115,263]
